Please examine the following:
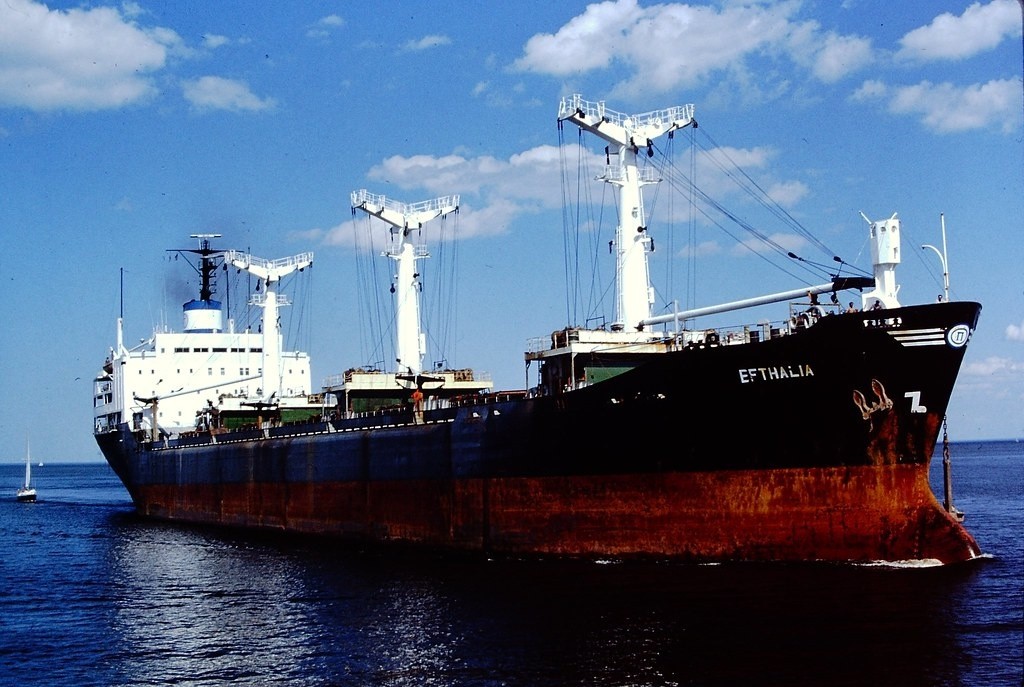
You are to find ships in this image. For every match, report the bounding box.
[87,89,1004,578]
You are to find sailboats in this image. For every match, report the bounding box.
[14,437,38,503]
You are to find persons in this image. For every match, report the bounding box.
[936,294,946,304]
[689,333,745,349]
[869,300,883,311]
[845,302,858,314]
[790,290,835,335]
[105,356,110,364]
